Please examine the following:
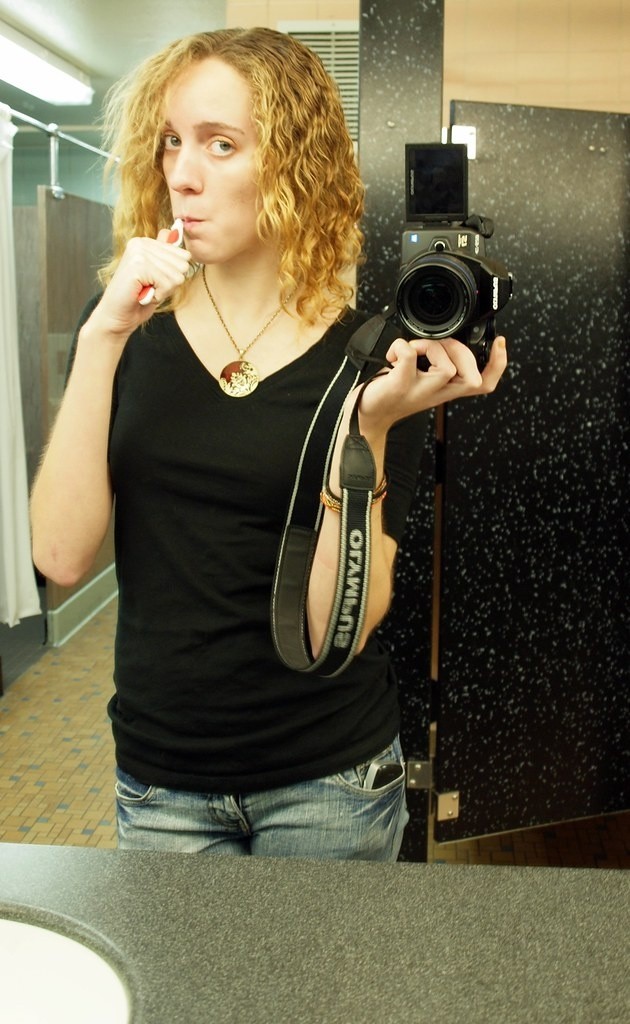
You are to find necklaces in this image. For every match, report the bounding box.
[199,265,296,397]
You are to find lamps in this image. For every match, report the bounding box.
[0,19,93,108]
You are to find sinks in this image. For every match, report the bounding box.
[1,915,133,1024]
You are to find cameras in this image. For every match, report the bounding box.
[393,142,518,377]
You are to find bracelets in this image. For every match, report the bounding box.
[323,474,388,512]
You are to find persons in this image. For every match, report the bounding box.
[30,27,509,862]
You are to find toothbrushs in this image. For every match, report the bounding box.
[139,218,186,305]
